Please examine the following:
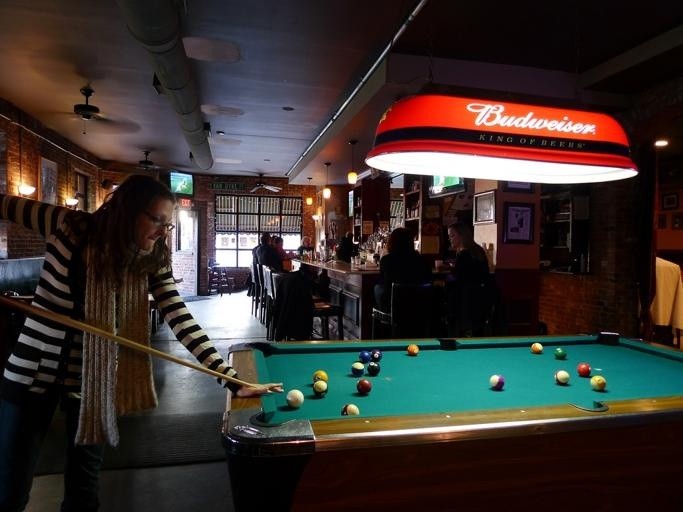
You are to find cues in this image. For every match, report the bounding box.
[0,295,273,395]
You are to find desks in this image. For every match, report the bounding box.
[221,330,683,511]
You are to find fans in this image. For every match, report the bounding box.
[39,88,141,136]
[125,151,178,174]
[244,176,282,194]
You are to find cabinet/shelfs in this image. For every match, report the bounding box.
[352,178,390,246]
[402,174,444,269]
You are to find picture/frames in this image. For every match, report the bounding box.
[37,155,59,206]
[363,218,374,237]
[503,201,534,245]
[656,192,683,230]
[500,181,535,194]
[472,188,497,224]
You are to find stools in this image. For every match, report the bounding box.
[209,266,231,297]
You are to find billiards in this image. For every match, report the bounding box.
[554,370,569,385]
[352,363,364,376]
[532,343,543,354]
[591,375,606,391]
[356,379,371,395]
[578,362,591,377]
[286,389,304,407]
[359,351,370,363]
[367,362,380,376]
[341,404,359,416]
[313,370,328,381]
[555,347,567,360]
[490,375,504,389]
[408,344,418,354]
[313,381,327,396]
[372,350,382,362]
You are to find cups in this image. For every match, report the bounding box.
[435,260,444,272]
[301,237,362,267]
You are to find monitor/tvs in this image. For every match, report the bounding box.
[168,171,194,197]
[428,174,465,199]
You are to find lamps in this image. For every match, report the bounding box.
[347,141,358,184]
[306,178,313,205]
[363,93,639,185]
[322,162,331,198]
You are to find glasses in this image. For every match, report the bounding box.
[139,210,175,231]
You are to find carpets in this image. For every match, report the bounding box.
[34,411,226,477]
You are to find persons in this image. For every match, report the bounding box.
[297,236,314,254]
[372,227,439,337]
[1,175,283,512]
[447,222,489,339]
[516,210,523,228]
[252,232,295,272]
[327,231,353,263]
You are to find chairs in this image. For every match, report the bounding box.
[250,258,345,340]
[370,281,433,339]
